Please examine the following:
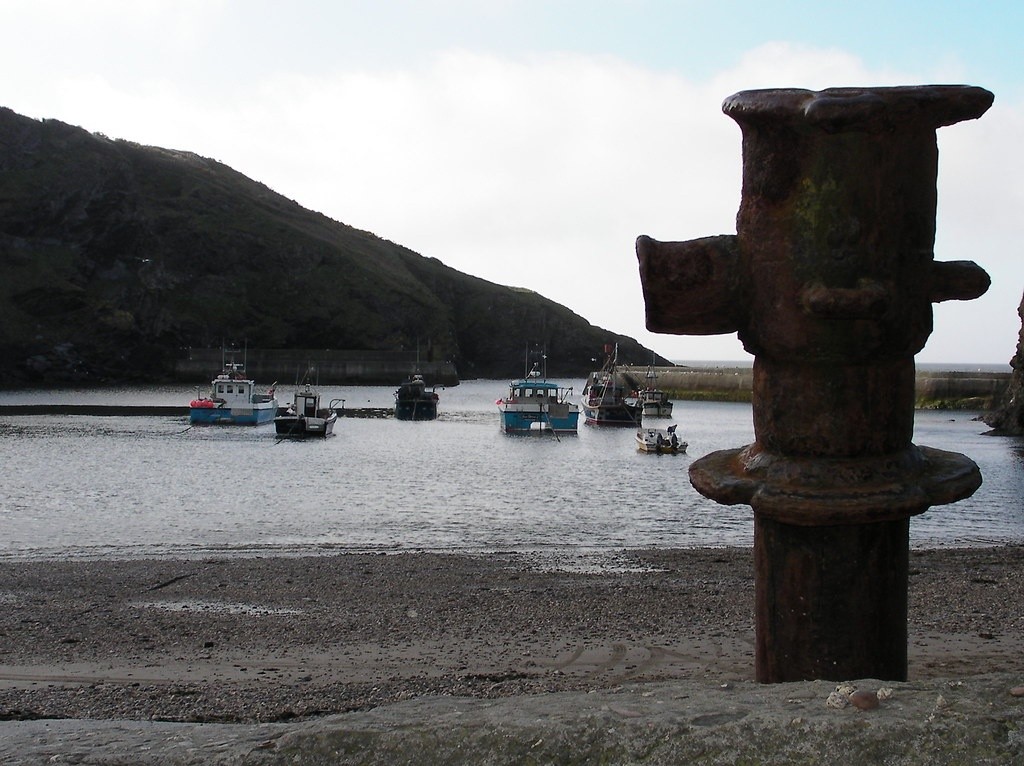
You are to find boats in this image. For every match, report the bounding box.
[392,368,445,423]
[637,364,674,418]
[634,425,688,456]
[580,343,643,427]
[495,344,579,434]
[188,342,278,425]
[274,383,339,440]
